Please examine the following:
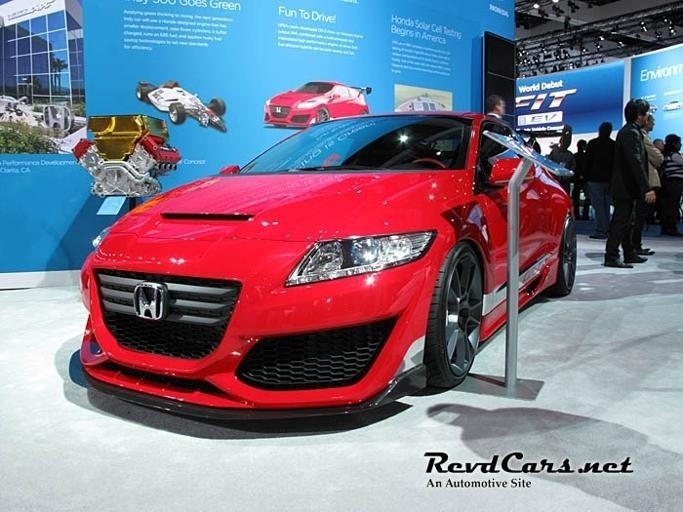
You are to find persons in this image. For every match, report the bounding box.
[485,93,682,270]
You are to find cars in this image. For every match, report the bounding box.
[136,80,228,132]
[81,110,578,423]
[264,82,370,129]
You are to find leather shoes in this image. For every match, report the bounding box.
[588,227,683,268]
